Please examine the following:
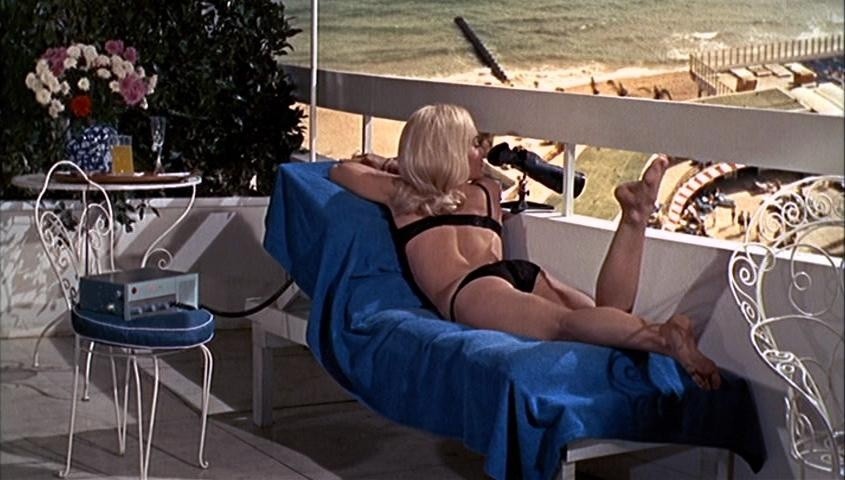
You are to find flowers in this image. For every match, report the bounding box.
[25,39,161,125]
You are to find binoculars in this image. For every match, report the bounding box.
[487,142,586,200]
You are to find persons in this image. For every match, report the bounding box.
[329,101,722,394]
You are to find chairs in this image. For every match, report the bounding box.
[35,159,222,478]
[239,161,763,480]
[724,169,845,480]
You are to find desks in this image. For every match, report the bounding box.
[7,169,204,404]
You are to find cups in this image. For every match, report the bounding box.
[108,135,135,176]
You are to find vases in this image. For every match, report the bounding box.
[64,119,121,175]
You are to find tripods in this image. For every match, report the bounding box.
[500,180,554,214]
